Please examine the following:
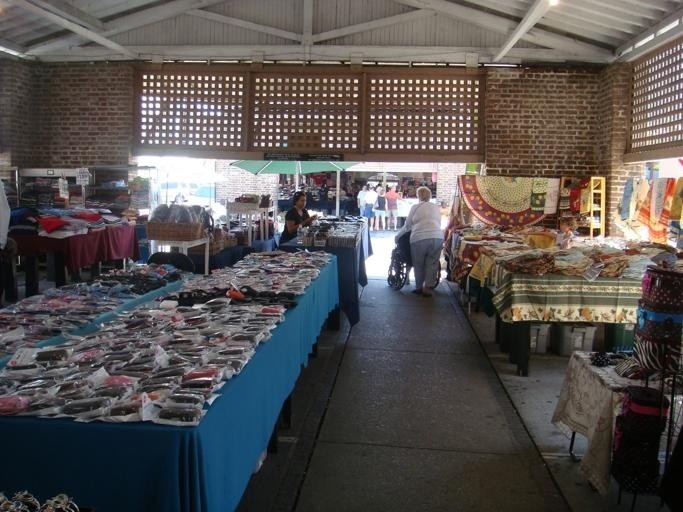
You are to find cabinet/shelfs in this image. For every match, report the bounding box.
[557,176,606,236]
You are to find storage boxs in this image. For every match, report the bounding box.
[529,321,637,357]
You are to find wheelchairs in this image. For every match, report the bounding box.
[385,229,444,293]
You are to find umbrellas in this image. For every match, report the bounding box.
[229,160,359,192]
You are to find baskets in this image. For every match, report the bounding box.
[147,221,201,240]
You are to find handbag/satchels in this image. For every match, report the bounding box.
[235,194,269,207]
[150,205,209,228]
[632,261,682,375]
[613,384,671,478]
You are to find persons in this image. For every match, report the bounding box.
[559,217,578,240]
[405,186,445,297]
[357,185,406,231]
[278,191,318,245]
[440,202,449,231]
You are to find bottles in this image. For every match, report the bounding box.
[327,191,333,201]
[296,225,302,244]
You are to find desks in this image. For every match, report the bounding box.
[444,224,557,315]
[552,351,683,511]
[0,185,372,510]
[481,252,642,375]
[372,198,437,222]
